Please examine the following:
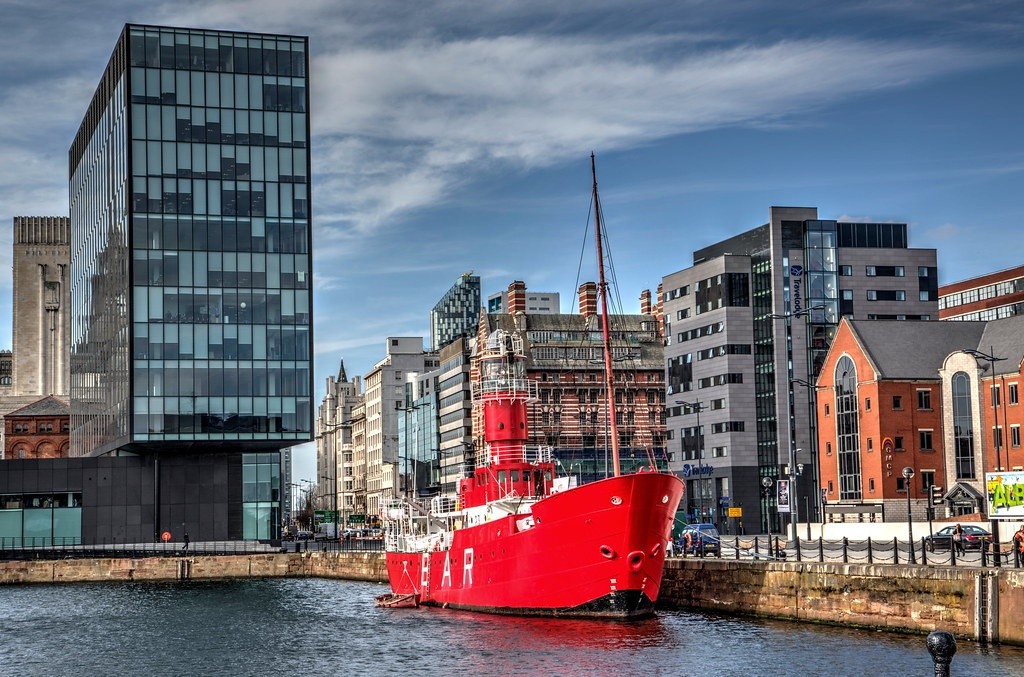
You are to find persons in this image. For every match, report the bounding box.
[1014,524,1024,568]
[951,523,966,558]
[302,537,309,553]
[344,530,350,549]
[180,532,191,554]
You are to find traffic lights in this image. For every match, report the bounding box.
[931,485,944,506]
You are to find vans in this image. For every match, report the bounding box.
[679,524,721,557]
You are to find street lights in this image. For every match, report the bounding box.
[378,440,473,504]
[902,466,918,565]
[394,405,409,509]
[961,345,1009,472]
[292,474,346,530]
[790,377,828,523]
[766,303,827,547]
[762,477,775,561]
[676,397,708,524]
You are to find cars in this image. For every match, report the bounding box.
[925,525,993,553]
[281,514,385,544]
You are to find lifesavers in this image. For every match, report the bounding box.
[681,532,693,548]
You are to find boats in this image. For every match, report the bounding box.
[366,148,686,624]
